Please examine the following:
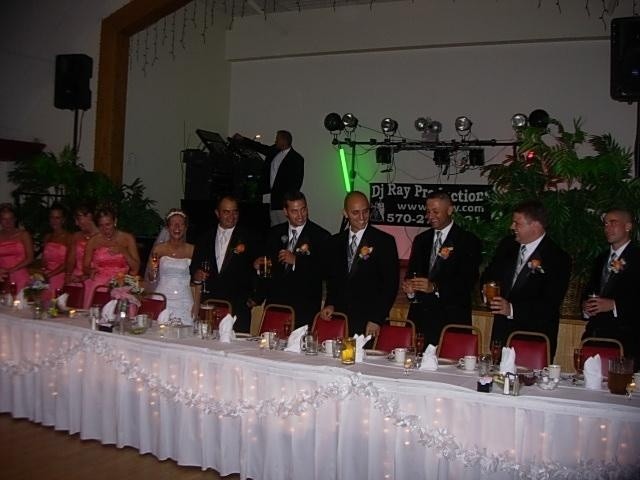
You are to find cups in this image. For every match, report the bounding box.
[259,255,273,279]
[2,280,635,396]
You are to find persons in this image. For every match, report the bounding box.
[400,192,482,353]
[144,208,202,322]
[255,190,332,332]
[81,208,140,310]
[64,201,100,310]
[189,193,262,334]
[579,210,640,373]
[234,131,304,227]
[320,191,400,348]
[0,203,34,296]
[479,200,571,366]
[41,204,75,298]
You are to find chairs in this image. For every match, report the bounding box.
[434,324,483,365]
[199,298,231,333]
[372,319,416,352]
[257,305,295,341]
[575,337,625,380]
[133,292,168,321]
[310,311,349,347]
[88,284,113,316]
[56,280,84,309]
[503,331,550,373]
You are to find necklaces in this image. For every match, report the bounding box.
[81,232,98,240]
[105,237,112,240]
[168,246,181,257]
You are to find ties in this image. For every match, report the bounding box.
[347,234,358,273]
[287,230,297,273]
[521,245,526,265]
[606,253,617,269]
[428,231,443,274]
[221,232,225,248]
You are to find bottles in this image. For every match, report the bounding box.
[404,270,417,302]
[151,251,158,282]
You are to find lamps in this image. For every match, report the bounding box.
[324,108,545,181]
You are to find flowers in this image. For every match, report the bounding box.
[357,243,375,261]
[229,238,248,257]
[525,258,546,274]
[435,244,456,261]
[293,241,311,256]
[605,254,629,276]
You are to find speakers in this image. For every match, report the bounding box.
[54,54,93,110]
[610,16,640,105]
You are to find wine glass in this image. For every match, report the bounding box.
[198,256,211,295]
